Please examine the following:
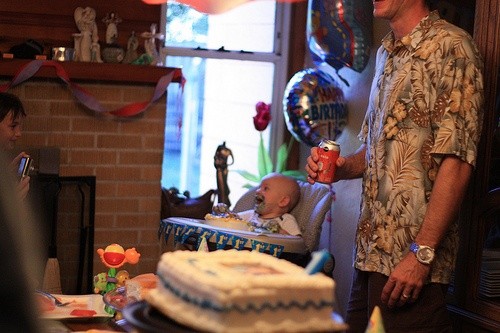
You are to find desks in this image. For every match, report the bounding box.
[37,295,126,333]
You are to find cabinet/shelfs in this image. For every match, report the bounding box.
[427,0,500,333]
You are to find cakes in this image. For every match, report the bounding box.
[204,213,246,230]
[149,249,335,333]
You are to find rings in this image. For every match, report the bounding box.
[402,293,410,299]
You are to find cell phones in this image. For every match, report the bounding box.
[17,155,32,182]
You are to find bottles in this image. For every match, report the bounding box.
[102,46,125,63]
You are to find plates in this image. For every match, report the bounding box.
[102,287,198,333]
[39,294,114,321]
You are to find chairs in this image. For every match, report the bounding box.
[157,178,334,275]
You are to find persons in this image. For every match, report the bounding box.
[104,11,119,45]
[143,23,160,66]
[305,0,484,333]
[212,173,304,238]
[0,92,35,200]
[77,7,94,62]
[128,31,140,63]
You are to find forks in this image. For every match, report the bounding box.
[38,291,74,306]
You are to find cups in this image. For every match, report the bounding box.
[52,47,73,62]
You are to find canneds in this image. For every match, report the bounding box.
[313,139,340,184]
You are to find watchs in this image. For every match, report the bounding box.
[409,242,436,265]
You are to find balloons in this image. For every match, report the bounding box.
[283,68,349,148]
[305,0,374,73]
